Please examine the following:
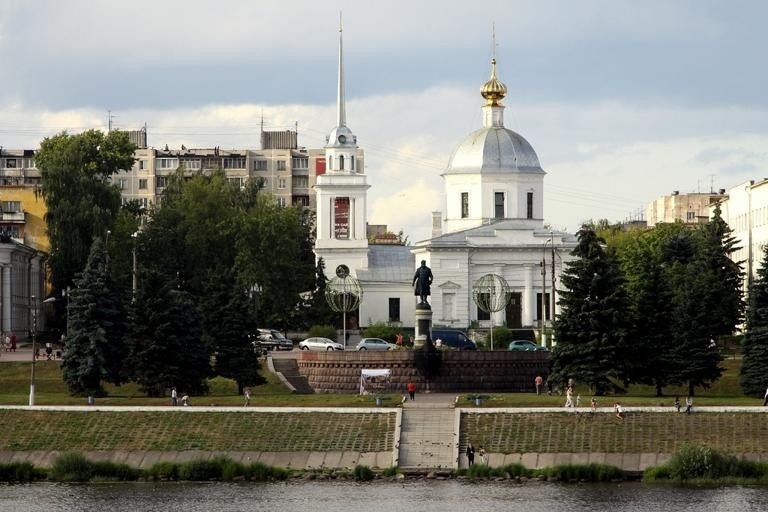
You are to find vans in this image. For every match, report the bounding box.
[248,328,293,351]
[431,330,478,350]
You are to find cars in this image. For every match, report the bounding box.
[509,340,546,351]
[356,338,398,351]
[299,337,344,351]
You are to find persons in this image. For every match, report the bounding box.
[674,393,693,414]
[478,446,485,464]
[88,393,94,405]
[395,332,414,345]
[466,442,475,467]
[4,331,66,360]
[407,380,416,401]
[615,402,624,420]
[534,373,598,411]
[763,385,768,406]
[244,389,252,407]
[172,387,177,406]
[436,337,443,347]
[412,260,433,304]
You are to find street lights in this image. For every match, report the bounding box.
[540,231,556,347]
[29,296,57,405]
[104,230,111,270]
[61,286,70,340]
[131,232,138,302]
